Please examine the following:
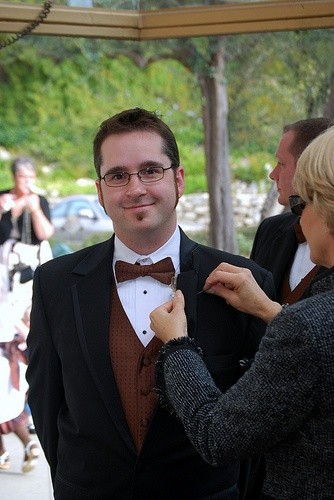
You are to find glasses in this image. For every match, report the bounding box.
[100,165,177,187]
[289,195,329,216]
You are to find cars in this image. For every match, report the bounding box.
[47,197,113,233]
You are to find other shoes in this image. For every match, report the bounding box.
[28,423,37,434]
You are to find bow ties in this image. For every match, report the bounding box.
[294,223,306,243]
[115,257,175,286]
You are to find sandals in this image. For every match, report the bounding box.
[21,441,40,473]
[0,451,11,469]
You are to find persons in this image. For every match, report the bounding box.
[148,124,334,500]
[247,115,334,306]
[0,156,55,293]
[24,107,276,500]
